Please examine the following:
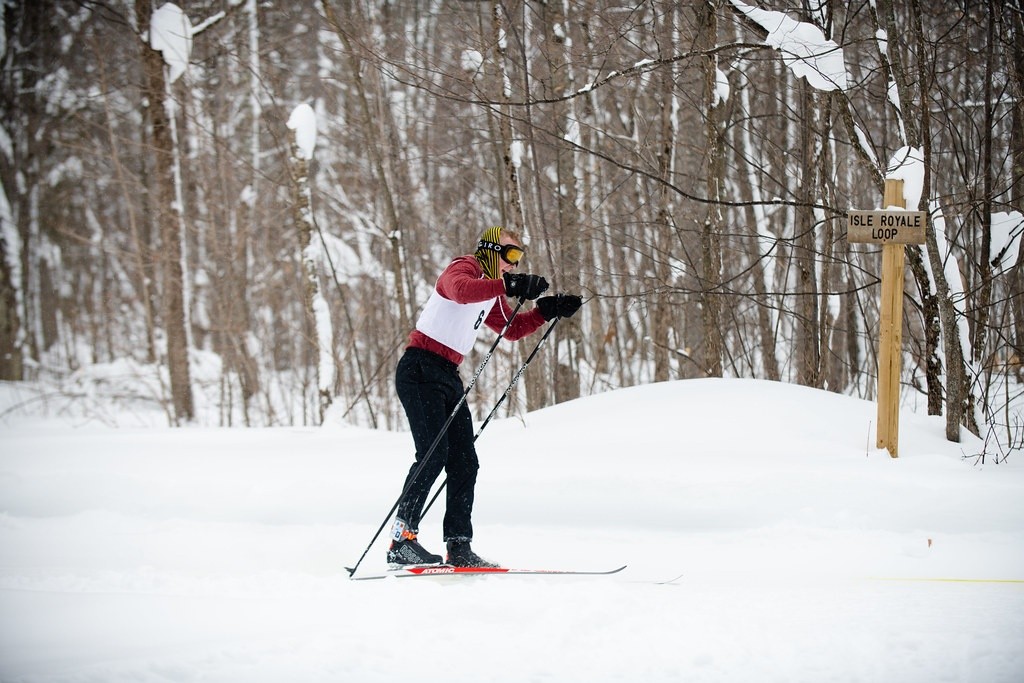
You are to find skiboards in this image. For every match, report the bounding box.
[345,565,627,582]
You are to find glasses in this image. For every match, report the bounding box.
[501,244,525,268]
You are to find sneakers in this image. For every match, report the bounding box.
[386,537,443,570]
[444,540,500,568]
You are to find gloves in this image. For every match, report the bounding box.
[503,272,549,301]
[536,293,583,322]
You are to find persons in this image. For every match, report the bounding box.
[387,226,582,564]
